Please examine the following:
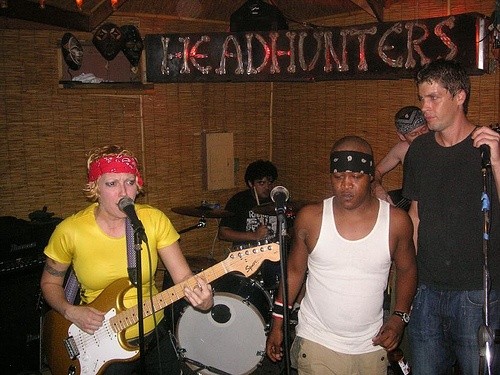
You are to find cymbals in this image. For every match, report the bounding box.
[185,255,219,272]
[171,206,229,218]
[252,201,320,216]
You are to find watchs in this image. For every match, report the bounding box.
[392,310,410,324]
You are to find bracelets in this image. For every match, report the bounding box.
[271,297,293,321]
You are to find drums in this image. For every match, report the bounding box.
[174,273,274,375]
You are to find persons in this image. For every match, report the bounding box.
[402,59,500,375]
[217,159,295,294]
[265,134,418,375]
[371,106,429,206]
[40,145,214,375]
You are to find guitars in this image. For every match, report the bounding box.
[41,237,282,375]
[386,123,500,213]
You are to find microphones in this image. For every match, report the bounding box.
[270,186,289,216]
[119,197,147,242]
[480,144,492,168]
